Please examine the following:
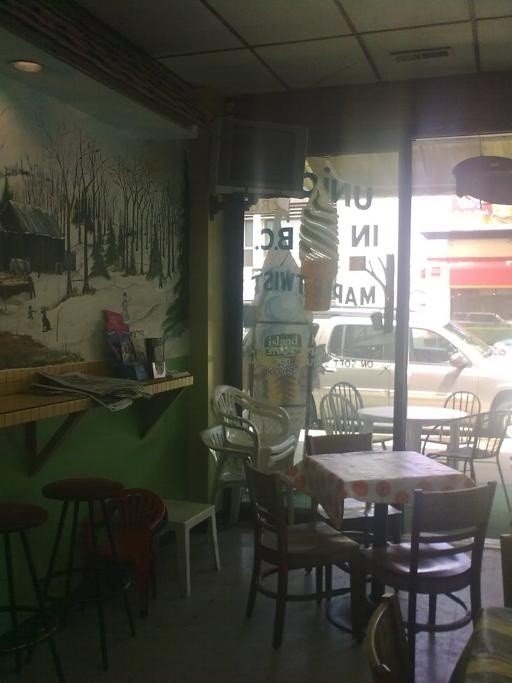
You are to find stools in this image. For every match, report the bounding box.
[1,477,136,682]
[167,500,221,598]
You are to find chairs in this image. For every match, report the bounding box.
[84,488,164,621]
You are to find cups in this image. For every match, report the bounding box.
[144,338,165,376]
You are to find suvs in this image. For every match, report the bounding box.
[311,317,511,434]
